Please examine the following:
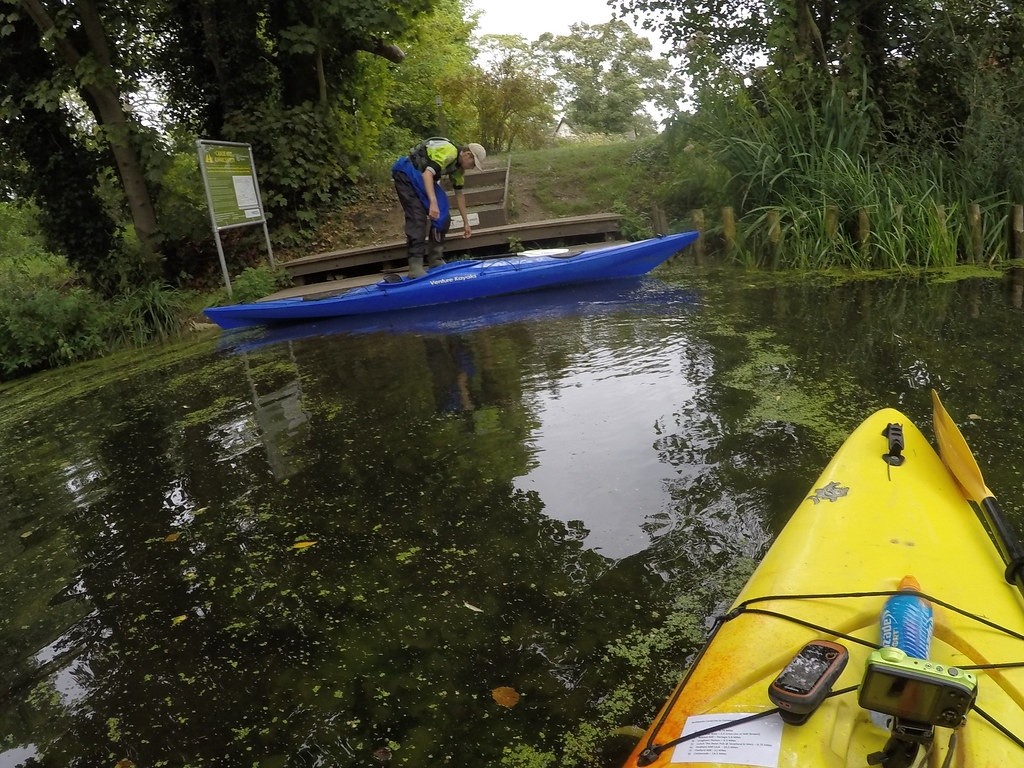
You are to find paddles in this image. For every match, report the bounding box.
[380,248,569,275]
[932,388,1024,599]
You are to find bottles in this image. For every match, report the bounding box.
[870,576,933,729]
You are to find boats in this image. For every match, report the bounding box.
[214,279,697,355]
[617,405,1024,767]
[204,231,700,330]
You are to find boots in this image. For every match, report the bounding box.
[428,245,447,267]
[408,255,428,279]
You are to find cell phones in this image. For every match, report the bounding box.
[767,639,849,716]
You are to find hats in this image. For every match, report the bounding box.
[468,142,486,174]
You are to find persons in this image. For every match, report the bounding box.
[392,137,486,279]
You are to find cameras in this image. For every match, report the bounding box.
[857,648,980,730]
